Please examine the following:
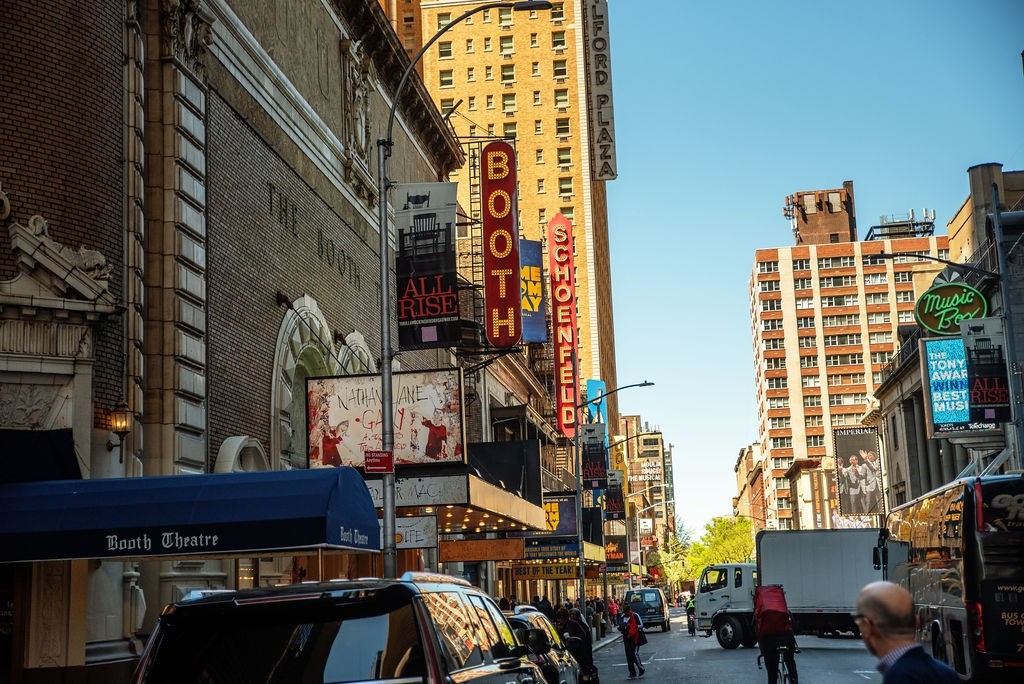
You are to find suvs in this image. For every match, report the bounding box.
[131,570,552,684]
[499,603,584,684]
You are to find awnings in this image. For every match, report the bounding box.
[0,467,380,565]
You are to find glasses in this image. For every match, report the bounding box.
[570,613,578,617]
[851,613,866,624]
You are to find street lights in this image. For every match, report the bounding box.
[573,381,663,611]
[603,431,663,637]
[375,0,555,580]
[635,501,674,589]
[624,483,673,590]
[870,252,1024,470]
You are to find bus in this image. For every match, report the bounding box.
[872,475,1024,684]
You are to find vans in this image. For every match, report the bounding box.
[623,588,672,633]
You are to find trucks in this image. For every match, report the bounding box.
[694,526,914,651]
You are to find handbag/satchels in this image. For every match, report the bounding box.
[638,628,648,647]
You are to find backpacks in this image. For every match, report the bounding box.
[751,584,790,621]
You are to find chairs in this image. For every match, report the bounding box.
[632,598,640,602]
[735,573,742,588]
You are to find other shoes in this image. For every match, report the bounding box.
[626,674,636,680]
[638,668,645,679]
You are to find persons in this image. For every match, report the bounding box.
[556,607,593,684]
[754,585,798,684]
[617,605,647,679]
[704,629,712,637]
[493,595,625,634]
[677,594,684,607]
[851,581,963,684]
[665,592,673,608]
[838,450,881,514]
[707,572,727,587]
[685,594,696,634]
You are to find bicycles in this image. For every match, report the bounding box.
[685,608,696,636]
[758,647,801,684]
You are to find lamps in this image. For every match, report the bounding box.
[106,400,133,464]
